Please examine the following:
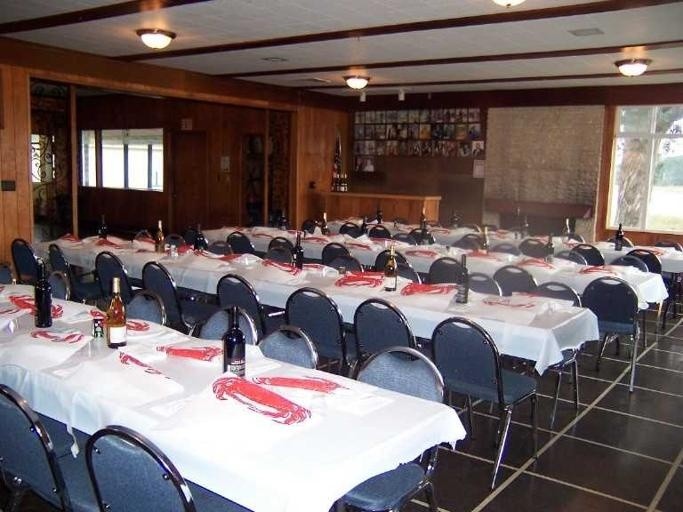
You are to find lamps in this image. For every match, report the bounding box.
[135,26,178,51]
[341,74,373,91]
[613,58,650,78]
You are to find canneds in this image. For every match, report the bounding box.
[92,316,104,338]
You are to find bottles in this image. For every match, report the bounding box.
[107,276,127,347]
[33,265,53,328]
[330,170,350,194]
[223,306,246,378]
[193,224,205,251]
[97,215,108,240]
[290,233,303,272]
[456,255,469,303]
[385,245,398,291]
[320,206,624,263]
[155,220,165,254]
